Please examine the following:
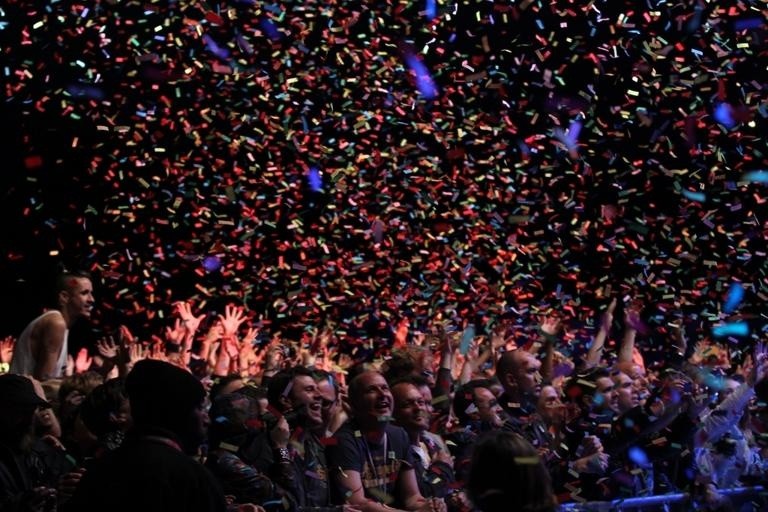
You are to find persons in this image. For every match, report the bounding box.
[2,268,768,511]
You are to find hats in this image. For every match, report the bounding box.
[125,359,205,426]
[1,373,52,409]
